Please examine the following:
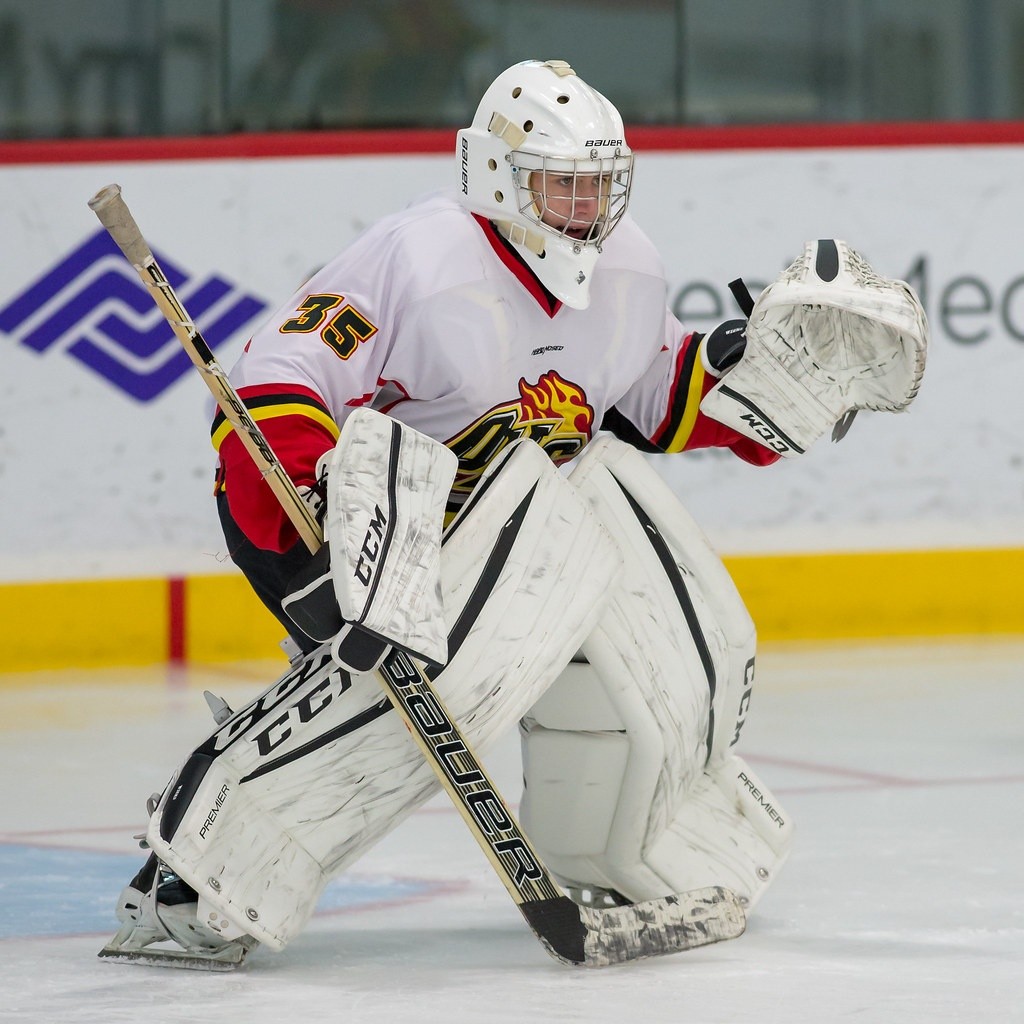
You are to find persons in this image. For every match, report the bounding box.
[117,60,927,950]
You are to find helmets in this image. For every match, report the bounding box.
[455,59,634,310]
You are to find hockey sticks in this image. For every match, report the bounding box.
[88,182,749,969]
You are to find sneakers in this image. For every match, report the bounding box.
[98,851,258,971]
